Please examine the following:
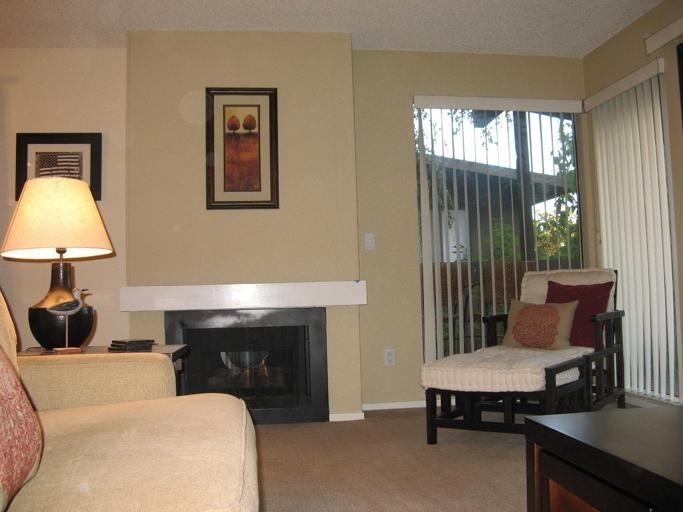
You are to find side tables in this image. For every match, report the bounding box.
[18,345,189,396]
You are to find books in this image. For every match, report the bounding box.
[106,339,159,351]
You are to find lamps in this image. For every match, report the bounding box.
[1,177,114,350]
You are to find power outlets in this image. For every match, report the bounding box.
[385,349,396,367]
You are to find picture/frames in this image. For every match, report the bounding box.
[205,88,279,209]
[15,133,102,203]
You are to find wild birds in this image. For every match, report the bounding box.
[46,287,88,316]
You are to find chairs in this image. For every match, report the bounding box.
[0,294,259,512]
[482,270,626,412]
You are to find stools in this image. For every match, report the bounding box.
[420,353,588,444]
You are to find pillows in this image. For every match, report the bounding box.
[0,349,44,511]
[503,269,616,350]
